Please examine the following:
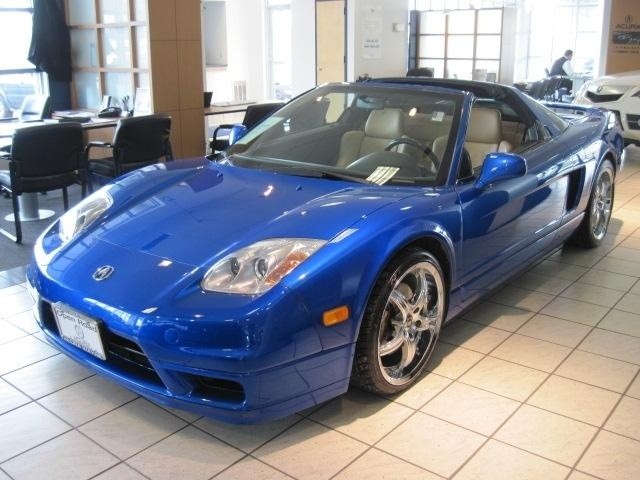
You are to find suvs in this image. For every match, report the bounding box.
[568,69,640,149]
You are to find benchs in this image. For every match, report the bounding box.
[401,115,526,170]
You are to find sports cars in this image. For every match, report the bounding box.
[26,69,628,428]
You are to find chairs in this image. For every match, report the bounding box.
[211,102,284,152]
[339,107,414,172]
[431,108,513,175]
[1,124,86,242]
[511,76,573,104]
[89,115,172,180]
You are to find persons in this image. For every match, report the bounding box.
[549,50,573,78]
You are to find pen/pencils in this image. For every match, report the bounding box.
[122,95,129,112]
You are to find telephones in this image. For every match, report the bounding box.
[98,107,121,118]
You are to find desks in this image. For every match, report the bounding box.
[1,110,119,224]
[203,105,257,128]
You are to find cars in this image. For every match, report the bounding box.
[0,73,48,122]
